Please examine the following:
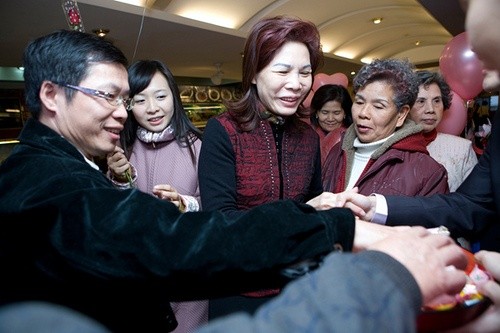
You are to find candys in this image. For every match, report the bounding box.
[424,267,491,310]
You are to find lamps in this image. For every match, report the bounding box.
[210,71,224,85]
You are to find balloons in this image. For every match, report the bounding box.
[434,91,467,135]
[438,29,487,100]
[311,72,349,92]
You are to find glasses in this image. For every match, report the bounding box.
[50,80,135,111]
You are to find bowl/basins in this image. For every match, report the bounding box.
[233,89,241,100]
[179,89,194,99]
[209,90,220,101]
[196,91,208,102]
[221,89,232,101]
[415,246,495,333]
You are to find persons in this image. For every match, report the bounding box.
[0,16,500,333]
